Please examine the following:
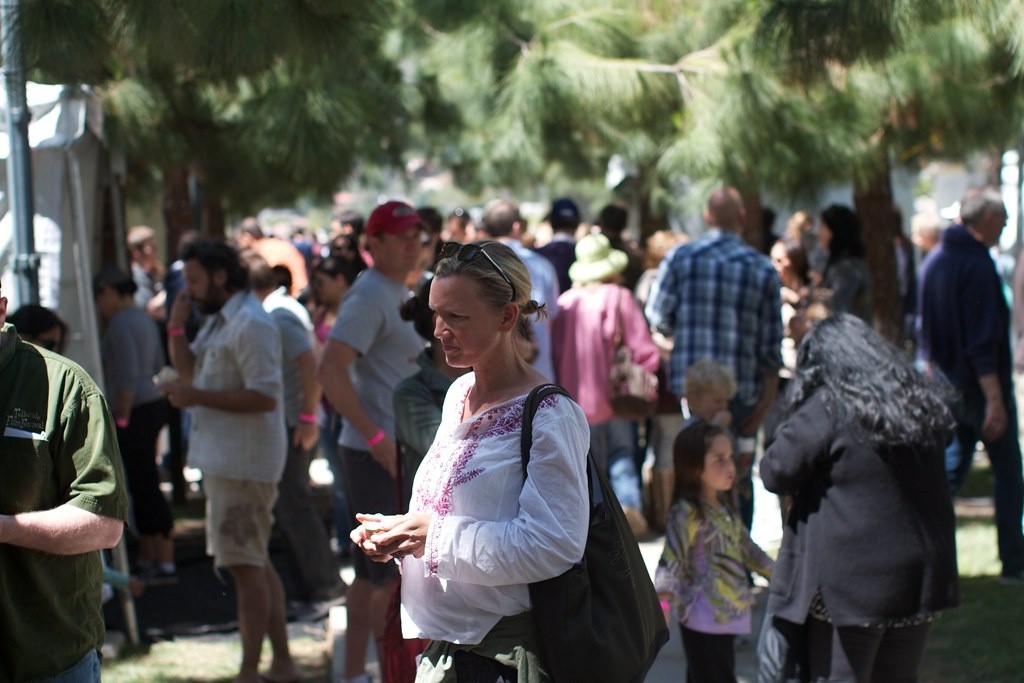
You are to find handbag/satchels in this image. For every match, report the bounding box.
[519,383,671,683]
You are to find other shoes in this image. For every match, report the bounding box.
[286,599,329,624]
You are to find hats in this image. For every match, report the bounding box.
[542,198,581,222]
[367,201,432,237]
[568,233,628,282]
[126,226,155,248]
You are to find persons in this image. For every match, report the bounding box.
[0,281,127,682]
[348,241,589,683]
[0,188,1024,683]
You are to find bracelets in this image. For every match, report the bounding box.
[661,601,670,611]
[367,430,384,447]
[116,418,128,428]
[299,412,316,421]
[169,327,186,335]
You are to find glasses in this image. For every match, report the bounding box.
[439,241,517,303]
[36,338,58,352]
